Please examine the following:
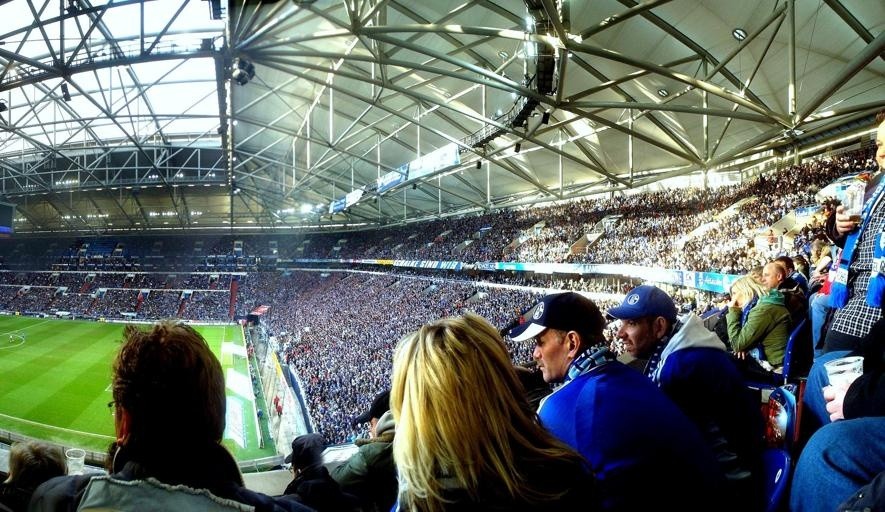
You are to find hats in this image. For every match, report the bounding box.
[606,285,678,323]
[507,290,605,343]
[283,431,327,465]
[355,389,390,423]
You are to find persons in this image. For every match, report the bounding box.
[0,445,66,511]
[330,390,394,485]
[661,285,730,314]
[838,473,885,512]
[584,298,629,358]
[775,256,809,296]
[508,292,726,511]
[607,286,770,510]
[750,266,764,283]
[263,163,763,275]
[726,275,791,384]
[809,273,828,293]
[826,126,885,354]
[0,236,263,323]
[763,144,879,261]
[401,276,483,332]
[790,316,885,512]
[388,313,599,512]
[484,275,549,371]
[810,240,832,278]
[29,325,314,512]
[762,262,808,325]
[548,276,636,292]
[264,275,370,441]
[283,435,339,512]
[810,250,842,348]
[370,277,401,400]
[794,255,809,281]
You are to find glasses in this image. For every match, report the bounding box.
[363,421,374,429]
[288,466,298,473]
[107,400,119,415]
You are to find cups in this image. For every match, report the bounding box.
[824,357,864,387]
[839,181,866,224]
[65,448,86,475]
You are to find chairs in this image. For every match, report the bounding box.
[747,317,807,393]
[764,448,792,511]
